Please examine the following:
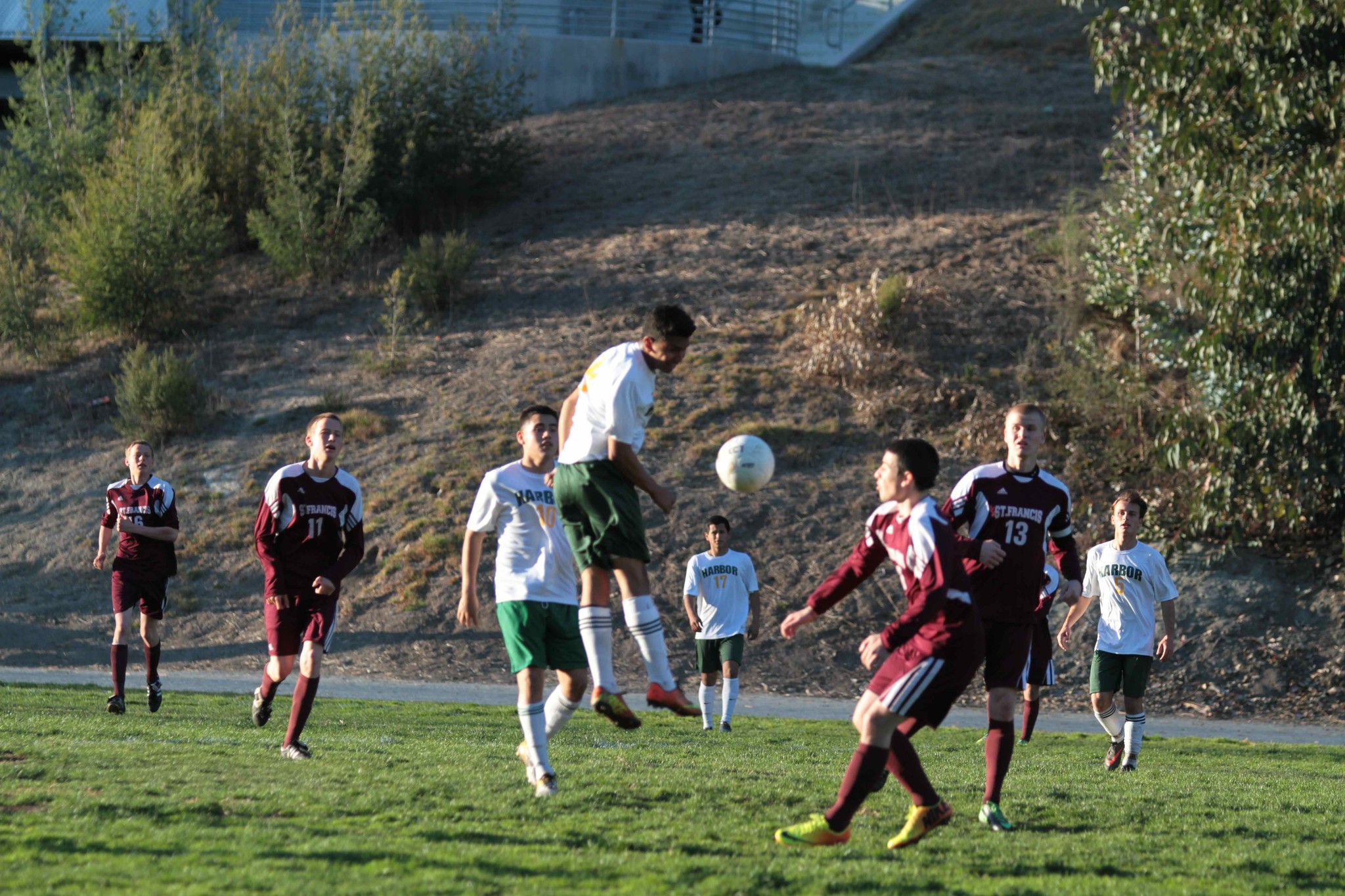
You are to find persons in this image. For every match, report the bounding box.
[458,406,588,794]
[870,404,1083,831]
[95,439,180,712]
[541,304,704,730]
[1057,490,1180,773]
[775,439,985,849]
[683,516,761,733]
[976,561,1058,748]
[252,414,365,758]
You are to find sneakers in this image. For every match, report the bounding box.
[533,772,559,798]
[1105,738,1124,771]
[251,685,273,728]
[108,695,127,713]
[281,740,311,761]
[644,681,704,717]
[888,795,954,850]
[1119,764,1136,775]
[1016,739,1030,746]
[774,814,853,847]
[974,733,988,746]
[147,675,162,712]
[517,738,535,787]
[977,801,1017,832]
[589,684,642,729]
[720,723,731,732]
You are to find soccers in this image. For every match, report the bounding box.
[712,435,780,492]
